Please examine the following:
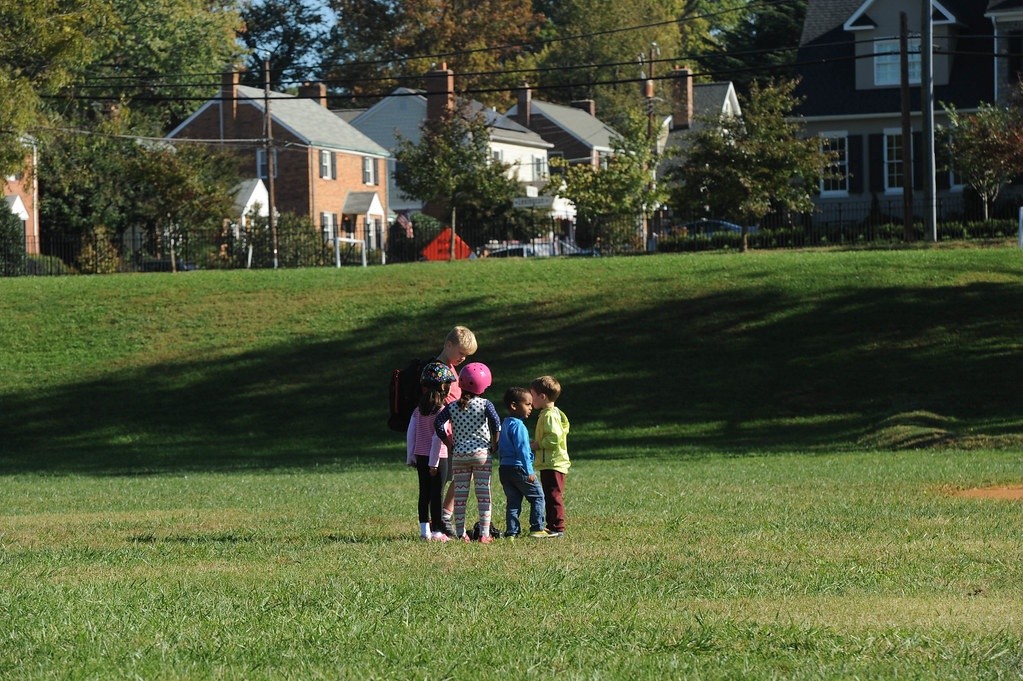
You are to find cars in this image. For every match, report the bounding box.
[487,246,548,258]
[548,240,600,258]
[666,220,761,237]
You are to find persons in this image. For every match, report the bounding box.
[406,326,478,542]
[531,376,571,537]
[498,387,548,538]
[434,363,501,544]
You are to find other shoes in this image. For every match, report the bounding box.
[420,535,431,542]
[505,536,514,541]
[474,521,500,539]
[530,530,558,538]
[478,533,494,544]
[440,517,457,538]
[458,534,470,542]
[544,528,563,536]
[431,532,450,543]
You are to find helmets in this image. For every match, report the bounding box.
[458,362,492,394]
[420,362,456,382]
